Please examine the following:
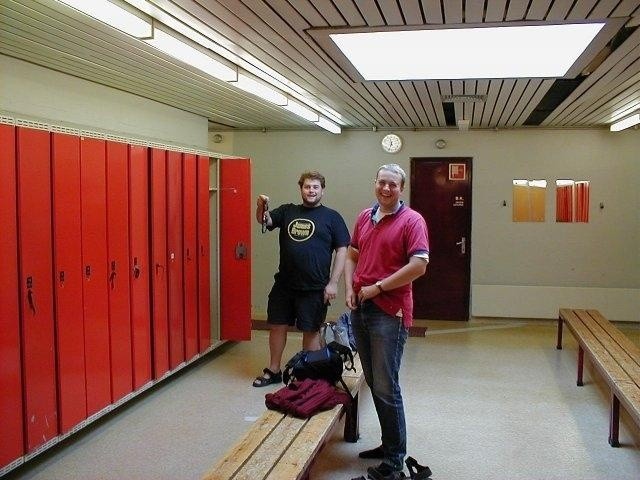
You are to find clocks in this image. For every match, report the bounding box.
[381,132,404,155]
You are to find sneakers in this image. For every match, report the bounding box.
[359,445,403,471]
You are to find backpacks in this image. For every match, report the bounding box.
[338,308,358,352]
[282,341,356,385]
[320,320,352,363]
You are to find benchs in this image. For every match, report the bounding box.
[557,308,640,448]
[201,375,361,479]
[341,347,366,388]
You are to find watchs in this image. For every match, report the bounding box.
[376,280,383,293]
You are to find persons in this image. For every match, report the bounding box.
[345,164,429,475]
[252,171,350,388]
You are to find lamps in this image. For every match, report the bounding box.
[609,109,640,132]
[58,0,341,136]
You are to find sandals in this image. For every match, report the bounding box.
[351,457,433,480]
[253,367,282,388]
